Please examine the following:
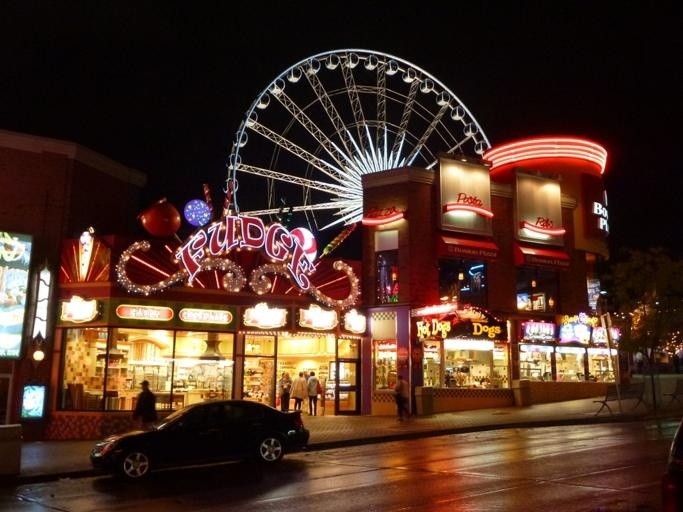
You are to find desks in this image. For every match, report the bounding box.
[133,390,184,410]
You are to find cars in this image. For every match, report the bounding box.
[89,397,312,482]
[659,418,683,510]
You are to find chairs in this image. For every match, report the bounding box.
[67,382,90,409]
[102,389,126,409]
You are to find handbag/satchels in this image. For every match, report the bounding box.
[316,380,323,394]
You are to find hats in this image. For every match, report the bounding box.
[139,380,149,386]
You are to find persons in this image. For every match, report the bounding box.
[392,375,413,423]
[130,380,158,430]
[279,370,319,416]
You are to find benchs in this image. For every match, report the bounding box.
[661,376,682,406]
[590,383,651,415]
